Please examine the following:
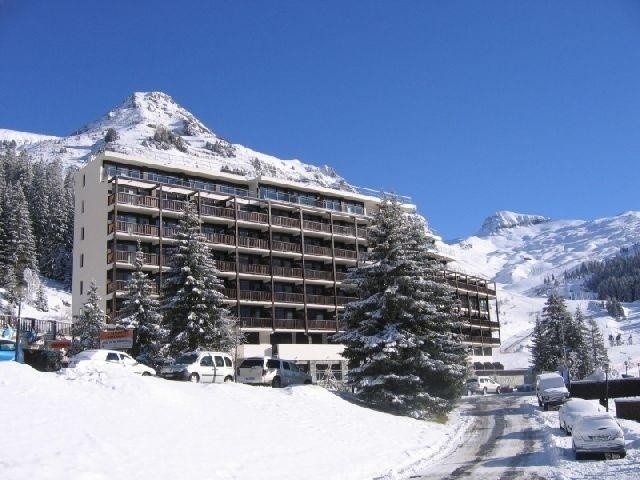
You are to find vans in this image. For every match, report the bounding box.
[173,350,235,383]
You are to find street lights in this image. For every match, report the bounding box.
[566,358,573,392]
[624,361,628,375]
[13,282,27,362]
[603,363,610,411]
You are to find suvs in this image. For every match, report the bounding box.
[536,374,569,411]
[236,356,312,388]
[467,376,501,395]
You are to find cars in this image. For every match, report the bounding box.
[69,349,157,377]
[559,400,626,460]
[0,340,24,364]
